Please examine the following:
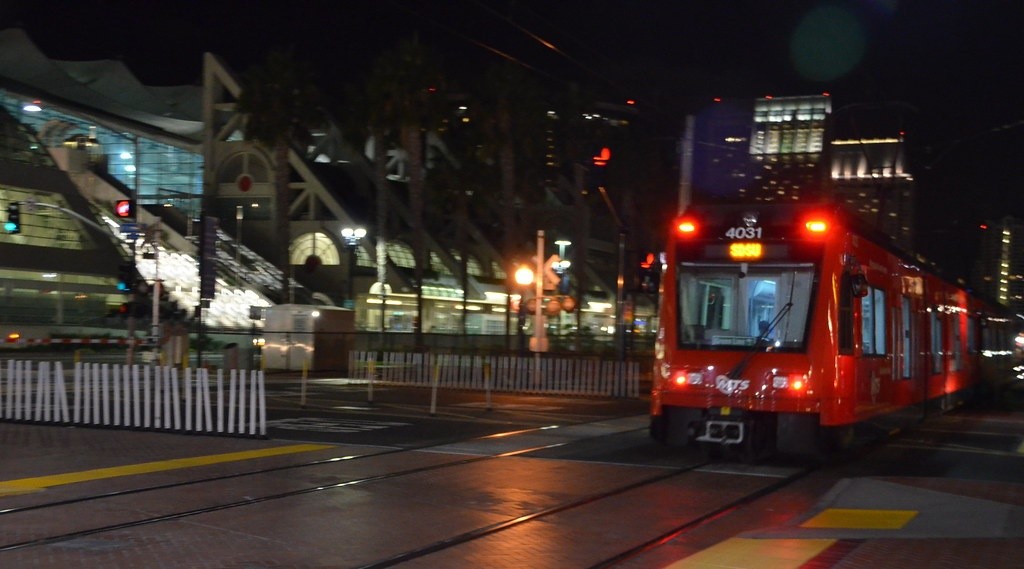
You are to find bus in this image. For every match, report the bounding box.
[651,197,1024,465]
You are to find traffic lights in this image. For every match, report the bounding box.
[116,200,135,219]
[8,203,19,233]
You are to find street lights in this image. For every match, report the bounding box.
[341,226,367,310]
[516,230,571,383]
